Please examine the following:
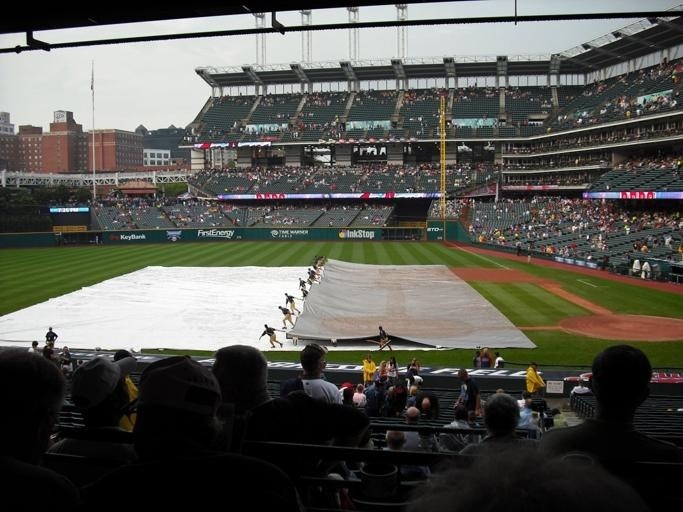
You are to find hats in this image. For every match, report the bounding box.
[139,356,223,417]
[72,356,137,410]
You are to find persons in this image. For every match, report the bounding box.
[459,60,682,271]
[2,326,682,512]
[88,88,463,229]
[259,256,324,348]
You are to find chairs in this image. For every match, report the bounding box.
[0,57,681,284]
[3,360,683,512]
